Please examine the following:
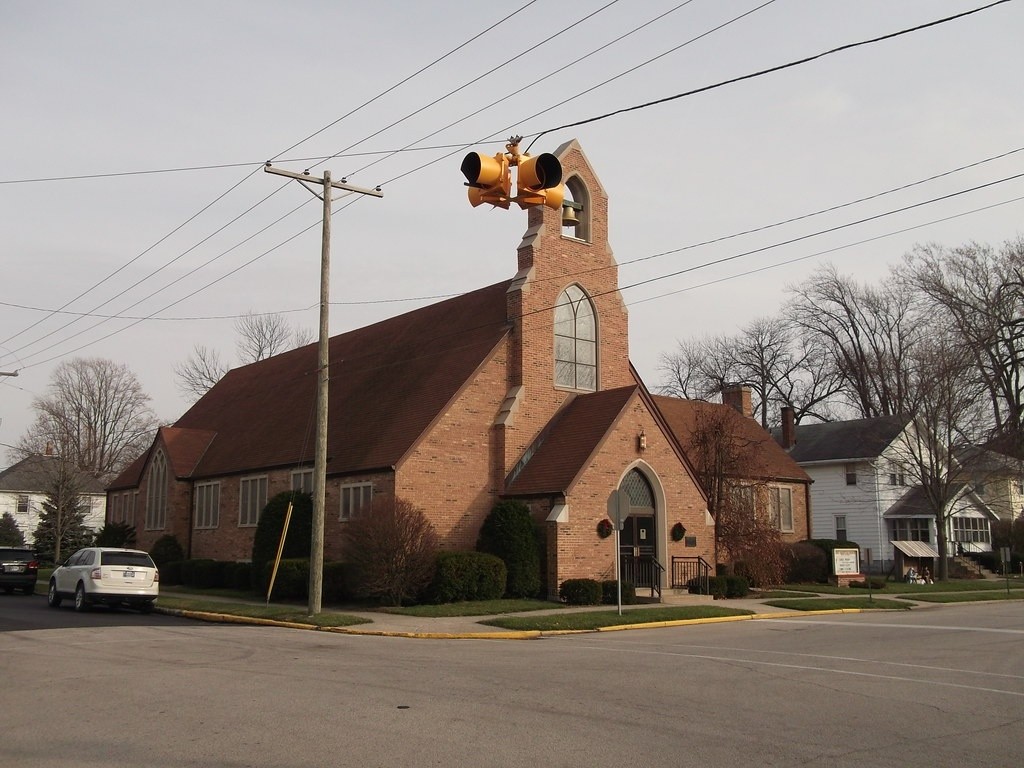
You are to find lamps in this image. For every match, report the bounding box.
[639,431,647,452]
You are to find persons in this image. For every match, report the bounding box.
[908,565,931,585]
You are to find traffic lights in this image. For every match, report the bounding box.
[458,152,512,211]
[514,150,566,212]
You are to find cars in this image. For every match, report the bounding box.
[0,545,40,594]
[48,546,160,614]
